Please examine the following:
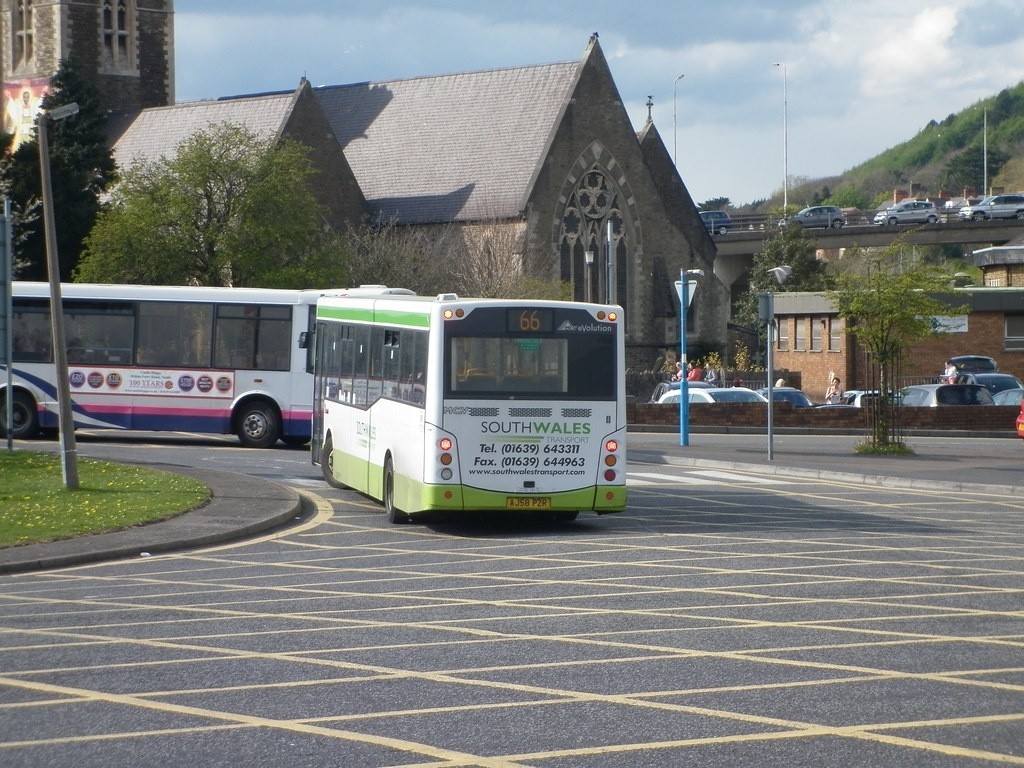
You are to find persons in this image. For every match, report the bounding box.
[775,379,785,387]
[825,377,844,404]
[942,359,968,384]
[672,361,717,388]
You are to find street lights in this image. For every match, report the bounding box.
[674,73,685,167]
[33,100,80,491]
[771,63,786,219]
[585,251,594,304]
[983,107,988,199]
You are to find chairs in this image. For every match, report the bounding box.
[232,349,289,371]
[407,360,562,392]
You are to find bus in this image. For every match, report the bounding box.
[0,280,322,449]
[299,286,628,525]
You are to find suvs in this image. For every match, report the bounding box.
[698,210,733,236]
[959,193,1024,222]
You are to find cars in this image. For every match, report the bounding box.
[813,355,1024,409]
[751,386,815,408]
[654,387,769,403]
[645,380,717,404]
[873,200,940,227]
[778,205,846,230]
